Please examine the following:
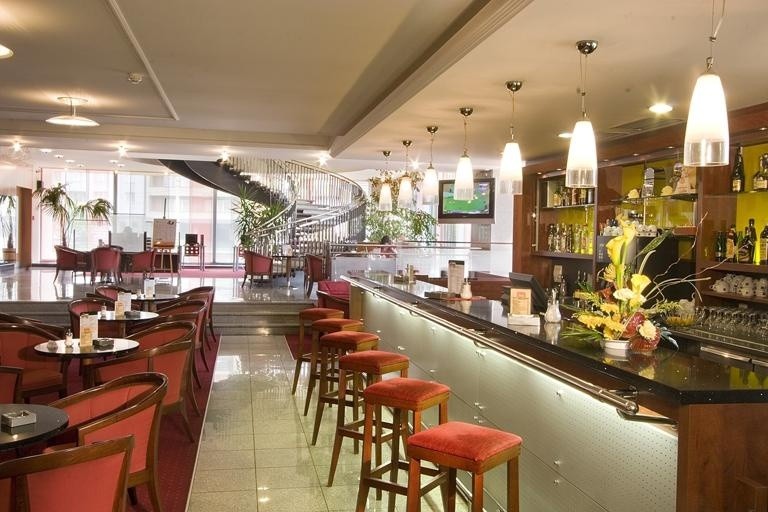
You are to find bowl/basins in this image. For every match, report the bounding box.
[602,220,657,237]
[708,272,768,298]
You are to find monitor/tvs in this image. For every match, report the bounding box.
[509,272,547,314]
[438,178,496,224]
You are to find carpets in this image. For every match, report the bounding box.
[283,334,348,361]
[83,265,246,278]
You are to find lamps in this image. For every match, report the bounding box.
[45,97,100,129]
[682,0,731,168]
[0,44,13,60]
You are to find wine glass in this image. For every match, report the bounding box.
[692,305,767,344]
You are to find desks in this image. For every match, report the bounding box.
[331,256,400,280]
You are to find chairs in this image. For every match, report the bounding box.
[316,281,350,320]
[305,253,326,298]
[241,250,274,289]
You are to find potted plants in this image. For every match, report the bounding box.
[0,194,16,263]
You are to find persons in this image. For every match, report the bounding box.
[373,235,398,258]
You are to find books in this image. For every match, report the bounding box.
[508,312,540,327]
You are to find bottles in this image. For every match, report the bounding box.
[560,279,567,296]
[729,144,748,195]
[101,302,106,318]
[573,270,592,309]
[714,218,767,264]
[551,184,594,207]
[751,153,767,191]
[460,278,473,300]
[64,329,74,346]
[544,221,593,256]
[543,300,561,323]
